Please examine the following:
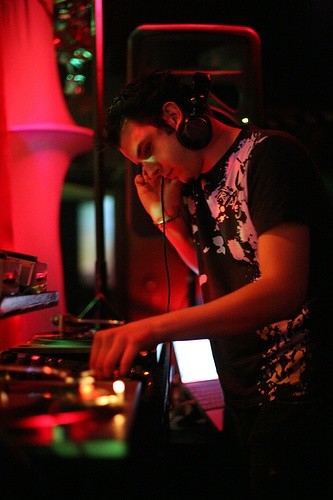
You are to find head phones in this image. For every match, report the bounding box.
[177,72,213,152]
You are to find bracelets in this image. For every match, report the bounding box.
[152,212,182,227]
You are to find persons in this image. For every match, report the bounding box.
[83,70,333,500]
